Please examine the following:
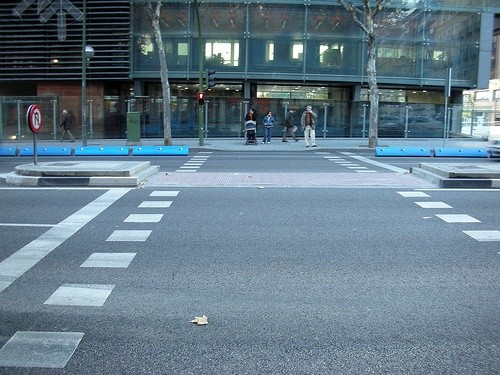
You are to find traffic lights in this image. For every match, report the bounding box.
[205,69,216,89]
[198,92,205,105]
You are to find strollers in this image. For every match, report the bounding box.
[244,120,259,145]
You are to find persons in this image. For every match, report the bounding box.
[245,109,256,142]
[282,110,299,142]
[59,110,75,142]
[301,105,317,146]
[263,111,275,144]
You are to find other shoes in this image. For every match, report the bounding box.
[306,145,310,147]
[267,141,271,144]
[282,141,289,142]
[312,145,319,147]
[262,142,265,144]
